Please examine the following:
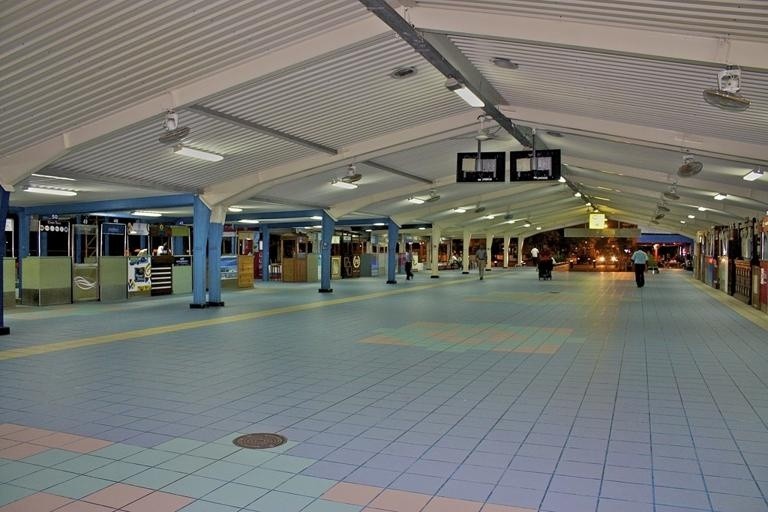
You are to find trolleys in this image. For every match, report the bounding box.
[537,259,553,281]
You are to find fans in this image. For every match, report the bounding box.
[677,155,703,178]
[651,203,672,225]
[425,189,532,229]
[701,66,750,113]
[663,184,680,200]
[342,165,361,183]
[159,110,189,145]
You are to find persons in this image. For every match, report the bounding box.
[475,244,487,280]
[404,248,413,280]
[493,254,499,267]
[531,245,540,266]
[630,246,648,287]
[448,250,463,269]
[539,244,554,259]
[157,243,172,256]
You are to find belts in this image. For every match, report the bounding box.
[479,258,485,261]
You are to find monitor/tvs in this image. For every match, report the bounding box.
[510,149,561,181]
[456,152,505,182]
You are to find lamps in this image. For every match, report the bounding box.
[741,169,762,182]
[173,146,223,162]
[445,77,486,108]
